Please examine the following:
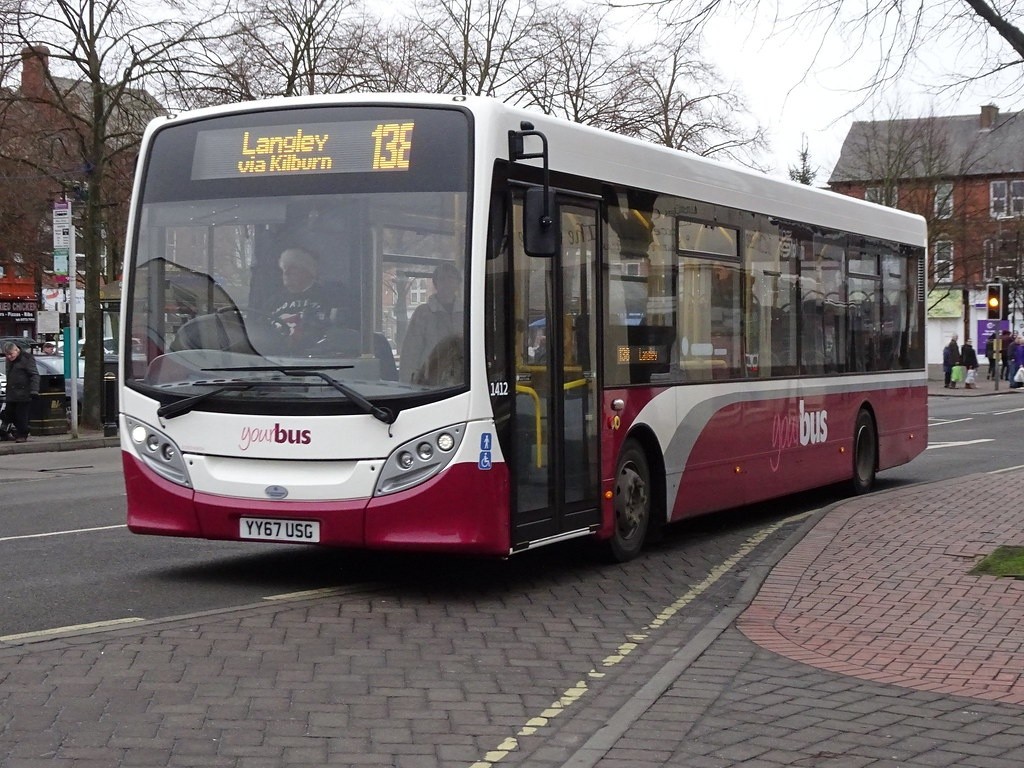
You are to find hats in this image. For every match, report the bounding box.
[1002,330,1011,335]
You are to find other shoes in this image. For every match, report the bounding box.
[992,377,996,380]
[1005,376,1009,380]
[966,385,974,389]
[1010,386,1020,388]
[1016,387,1024,389]
[1000,374,1003,379]
[16,433,31,442]
[945,385,951,388]
[987,373,991,379]
[949,385,959,389]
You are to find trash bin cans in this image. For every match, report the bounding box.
[28,374,68,436]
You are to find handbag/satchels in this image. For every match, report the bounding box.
[951,365,963,382]
[964,369,975,383]
[1014,367,1024,382]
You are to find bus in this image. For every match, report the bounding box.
[110,90,932,575]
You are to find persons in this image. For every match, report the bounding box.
[960,337,979,389]
[984,329,1024,388]
[398,261,465,386]
[252,246,353,359]
[943,332,961,389]
[41,343,55,356]
[1,340,41,444]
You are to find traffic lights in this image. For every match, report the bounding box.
[986,283,1003,321]
[1002,281,1013,321]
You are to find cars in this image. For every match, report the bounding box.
[0,333,148,441]
[744,348,830,378]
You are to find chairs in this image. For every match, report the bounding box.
[225,286,908,399]
